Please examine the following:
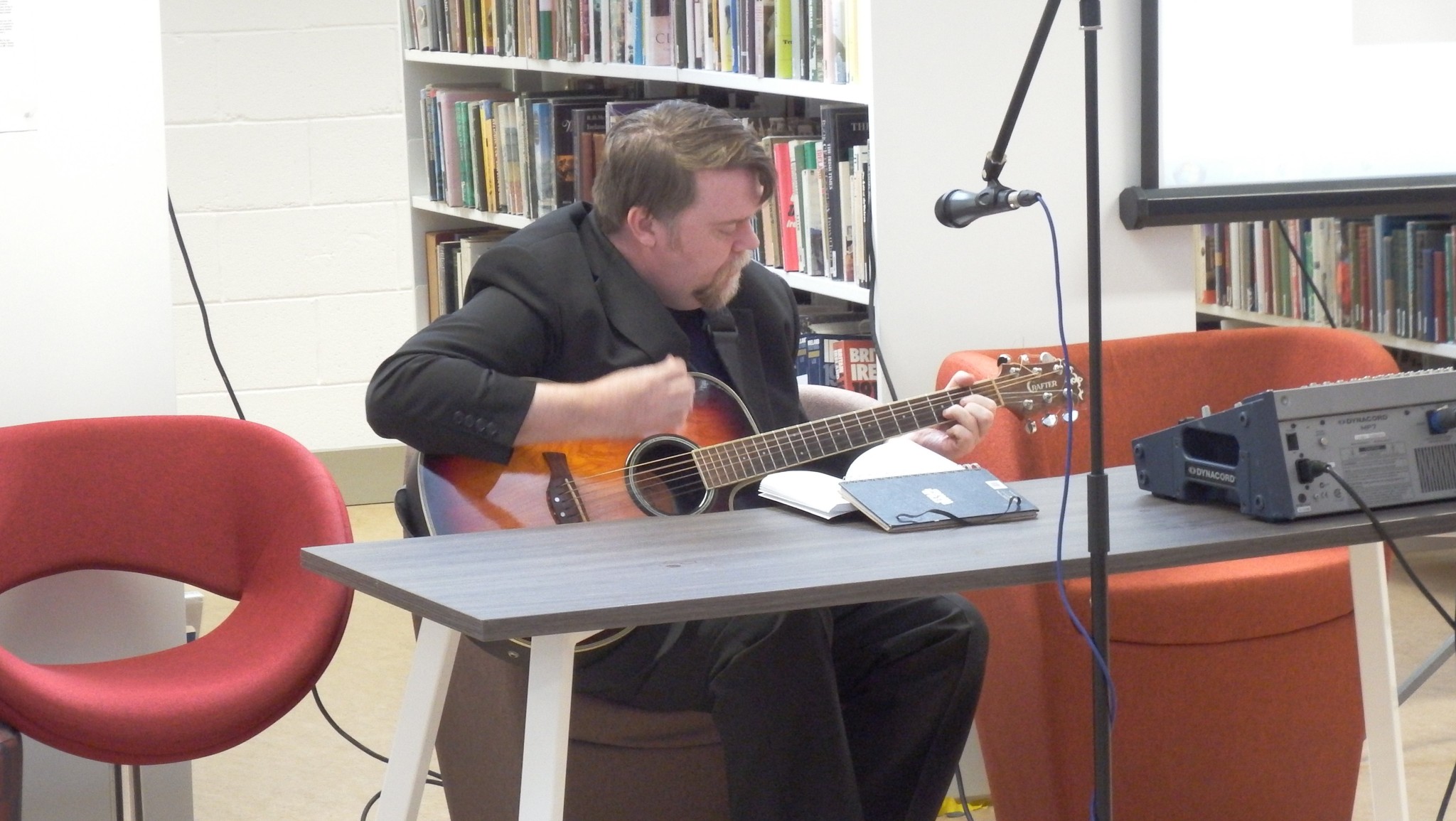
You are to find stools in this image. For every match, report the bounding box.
[0,414,354,821]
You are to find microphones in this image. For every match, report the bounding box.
[934,189,1042,228]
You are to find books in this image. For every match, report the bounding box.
[757,439,965,520]
[408,0,879,397]
[1193,214,1456,371]
[836,461,1039,534]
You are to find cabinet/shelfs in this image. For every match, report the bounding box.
[1198,216,1456,371]
[399,52,879,405]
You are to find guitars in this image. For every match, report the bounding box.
[403,331,1090,669]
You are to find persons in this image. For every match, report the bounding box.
[366,97,998,821]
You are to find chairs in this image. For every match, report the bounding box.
[935,327,1401,820]
[398,450,734,821]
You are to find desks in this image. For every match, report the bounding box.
[303,464,1456,821]
